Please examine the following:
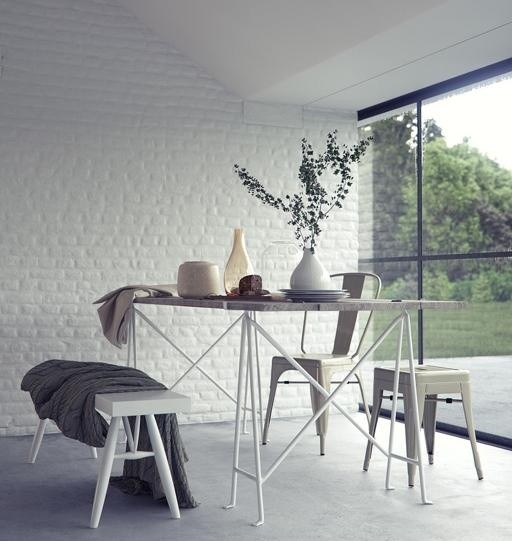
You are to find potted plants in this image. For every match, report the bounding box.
[232,129,375,292]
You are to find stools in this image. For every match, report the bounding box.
[362,363,484,489]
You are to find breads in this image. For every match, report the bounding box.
[239,275,262,295]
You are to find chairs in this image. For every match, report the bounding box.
[261,271,383,457]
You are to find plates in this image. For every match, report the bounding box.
[208,292,271,300]
[278,289,351,303]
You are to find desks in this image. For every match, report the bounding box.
[123,297,471,530]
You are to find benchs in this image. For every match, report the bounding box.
[26,358,193,530]
